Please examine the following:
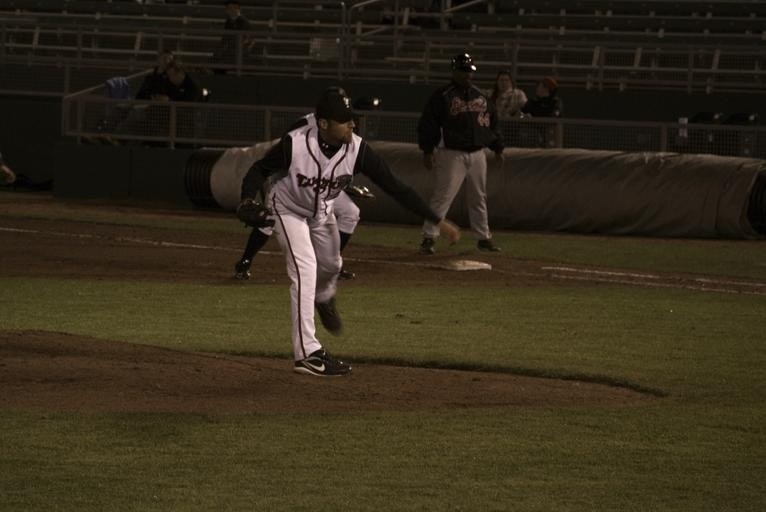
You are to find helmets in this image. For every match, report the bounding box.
[450,53,477,72]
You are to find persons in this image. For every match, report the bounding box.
[238,88,462,377]
[484,68,532,120]
[521,77,561,148]
[417,53,502,256]
[221,4,249,76]
[233,185,376,279]
[135,52,194,146]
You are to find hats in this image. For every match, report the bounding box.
[312,94,365,123]
[540,76,559,92]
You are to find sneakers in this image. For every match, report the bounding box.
[336,267,356,281]
[294,348,352,379]
[314,297,343,338]
[417,238,436,256]
[234,259,251,280]
[477,238,502,252]
